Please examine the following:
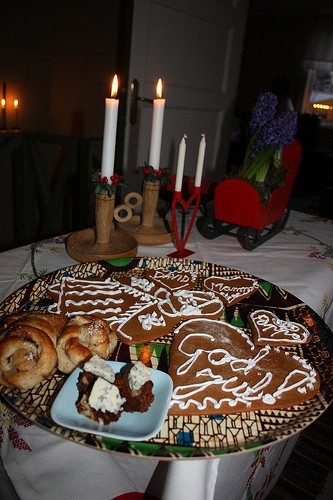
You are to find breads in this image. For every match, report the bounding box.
[0,310,118,390]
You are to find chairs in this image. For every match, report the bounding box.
[0,130,81,251]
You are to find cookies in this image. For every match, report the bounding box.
[47,267,321,416]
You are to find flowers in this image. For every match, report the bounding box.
[237,90,299,181]
[131,160,173,187]
[89,168,123,199]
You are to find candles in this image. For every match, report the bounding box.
[174,133,188,191]
[13,99,19,129]
[194,133,207,188]
[147,77,166,172]
[1,98,7,131]
[100,73,120,185]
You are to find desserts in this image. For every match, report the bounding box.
[75,355,155,425]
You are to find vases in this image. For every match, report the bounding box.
[195,137,304,252]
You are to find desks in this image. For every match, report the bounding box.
[0,206,333,500]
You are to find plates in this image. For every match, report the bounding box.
[50,360,174,442]
